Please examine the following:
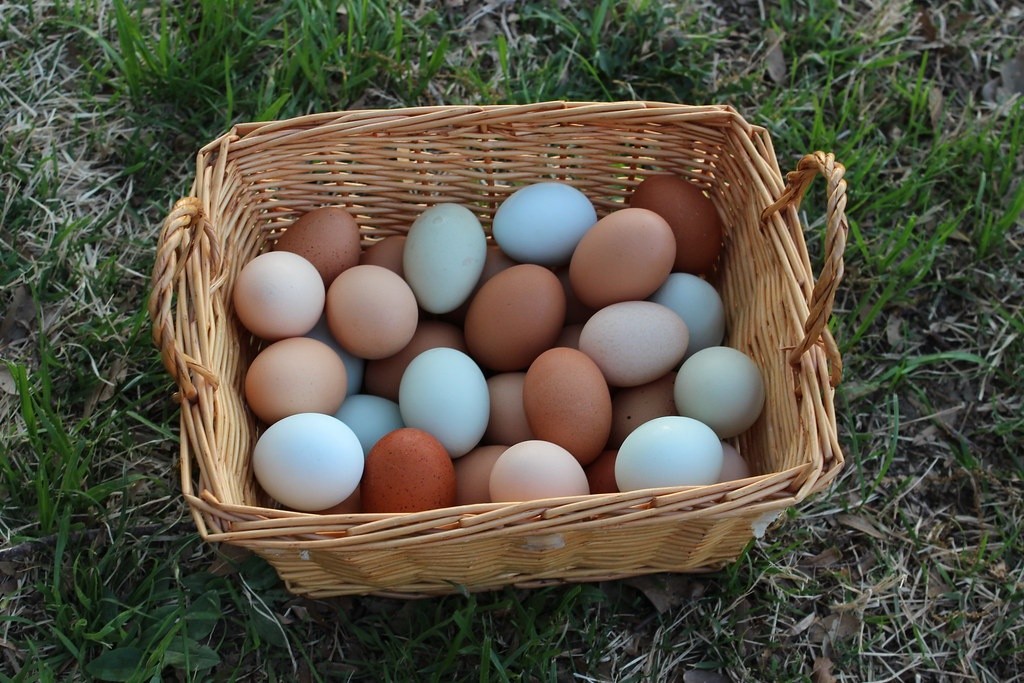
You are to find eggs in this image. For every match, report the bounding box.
[234,175,764,513]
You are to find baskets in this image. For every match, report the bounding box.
[149,104,848,598]
